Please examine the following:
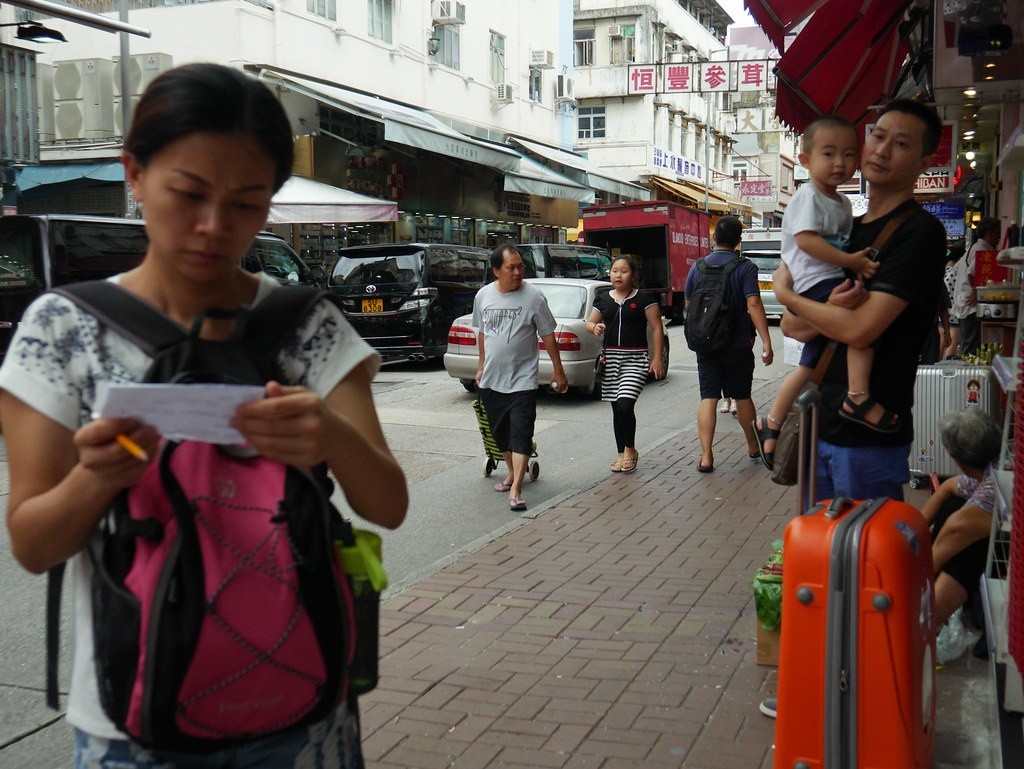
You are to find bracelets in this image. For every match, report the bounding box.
[593,324,597,333]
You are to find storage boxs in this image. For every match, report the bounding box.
[756,617,779,666]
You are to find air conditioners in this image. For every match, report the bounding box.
[37,53,173,145]
[608,25,622,37]
[670,43,682,54]
[557,74,575,100]
[432,0,465,25]
[497,83,512,102]
[531,50,552,69]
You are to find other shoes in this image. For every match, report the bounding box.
[720,400,731,413]
[759,698,776,719]
[730,400,738,415]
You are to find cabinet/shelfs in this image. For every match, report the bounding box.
[299,223,348,277]
[978,352,1024,714]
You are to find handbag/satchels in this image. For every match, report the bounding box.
[770,399,807,486]
[592,353,606,383]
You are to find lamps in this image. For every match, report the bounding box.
[0,19,68,43]
[299,119,377,159]
[429,38,440,56]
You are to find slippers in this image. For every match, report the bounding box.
[621,449,639,473]
[611,455,624,472]
[749,451,761,458]
[494,481,512,492]
[508,497,527,511]
[697,455,714,473]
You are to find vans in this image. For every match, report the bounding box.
[1,214,325,358]
[325,244,493,363]
[516,244,614,279]
[742,250,786,317]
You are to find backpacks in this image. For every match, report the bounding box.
[48,276,381,759]
[684,255,746,354]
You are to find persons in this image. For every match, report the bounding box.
[721,398,737,415]
[683,216,774,471]
[585,255,665,473]
[757,97,947,720]
[940,218,1000,362]
[1,64,410,768]
[751,118,901,470]
[919,409,1016,631]
[471,245,568,511]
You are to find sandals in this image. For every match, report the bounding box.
[839,393,900,433]
[752,417,781,471]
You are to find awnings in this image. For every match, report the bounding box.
[267,177,398,223]
[743,0,828,56]
[688,182,752,215]
[772,0,913,169]
[505,151,595,204]
[259,69,522,172]
[512,138,650,201]
[650,176,728,213]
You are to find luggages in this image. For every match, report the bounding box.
[908,359,1000,490]
[774,389,937,769]
[471,382,539,481]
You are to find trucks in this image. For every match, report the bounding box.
[582,200,710,325]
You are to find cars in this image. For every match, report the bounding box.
[443,278,670,401]
